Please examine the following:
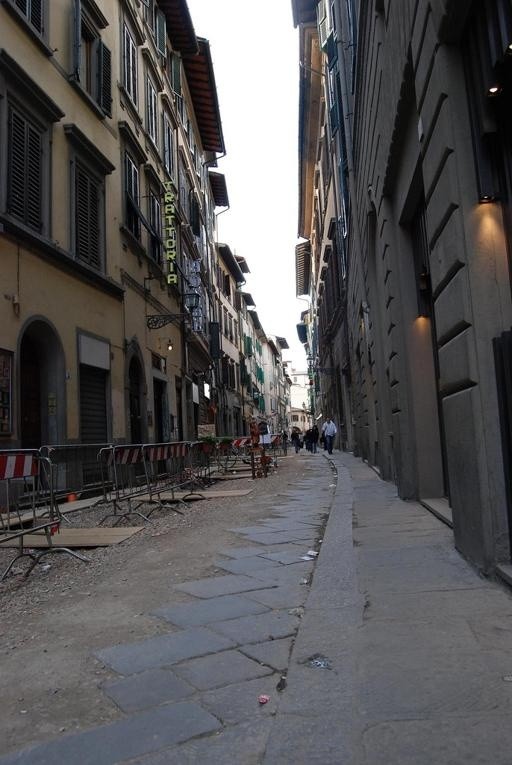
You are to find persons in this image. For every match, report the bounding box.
[321,417,337,454]
[282,431,287,439]
[321,431,328,450]
[290,425,319,454]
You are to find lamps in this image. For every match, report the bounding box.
[145,284,201,329]
